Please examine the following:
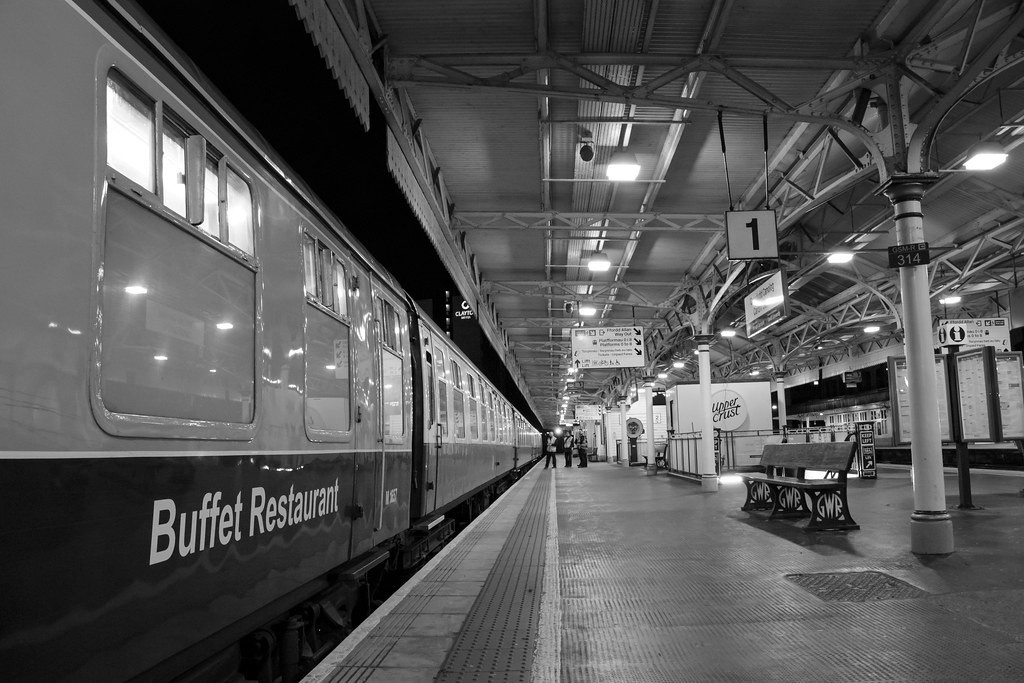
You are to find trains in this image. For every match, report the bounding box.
[0,0,545,683]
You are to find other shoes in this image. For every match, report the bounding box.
[552,466,556,468]
[577,465,586,468]
[565,464,571,467]
[544,466,547,469]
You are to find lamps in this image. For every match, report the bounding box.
[606,151,641,181]
[963,141,1010,172]
[587,252,611,272]
[578,301,596,316]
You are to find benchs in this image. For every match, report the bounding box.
[735,441,860,532]
[642,443,668,471]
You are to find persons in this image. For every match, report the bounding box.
[544,431,558,469]
[562,431,574,467]
[575,432,588,468]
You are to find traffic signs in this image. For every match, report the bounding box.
[571,326,643,366]
